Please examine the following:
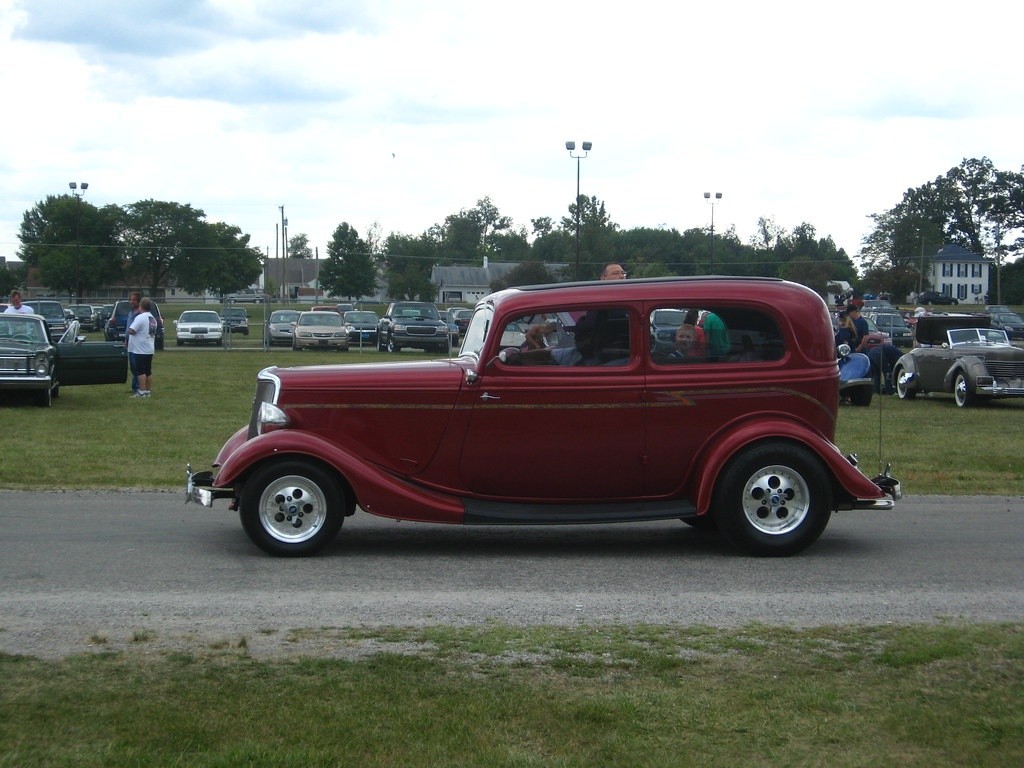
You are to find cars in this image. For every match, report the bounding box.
[183,277,902,558]
[831,290,1024,344]
[0,312,130,406]
[891,316,1024,407]
[835,345,875,404]
[225,287,267,305]
[68,304,112,332]
[436,306,689,347]
[263,302,382,345]
[174,311,225,346]
[0,304,12,314]
[291,311,351,352]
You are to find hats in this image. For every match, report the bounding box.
[912,306,926,318]
[844,304,857,313]
[838,311,847,318]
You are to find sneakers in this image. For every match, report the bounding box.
[129,390,152,399]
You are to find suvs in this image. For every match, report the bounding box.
[103,300,164,349]
[377,301,449,355]
[220,308,251,334]
[21,301,74,342]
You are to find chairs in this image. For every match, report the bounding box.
[420,308,428,315]
[304,317,314,324]
[395,308,403,315]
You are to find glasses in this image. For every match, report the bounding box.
[608,271,627,277]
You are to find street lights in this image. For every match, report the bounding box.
[705,193,722,275]
[566,140,593,280]
[68,181,90,303]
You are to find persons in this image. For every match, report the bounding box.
[3,290,35,315]
[698,312,708,328]
[565,315,608,368]
[683,309,706,357]
[845,304,869,356]
[124,292,142,394]
[702,313,731,363]
[867,344,907,393]
[834,311,857,359]
[586,262,629,348]
[127,297,158,399]
[520,322,564,350]
[667,324,697,358]
